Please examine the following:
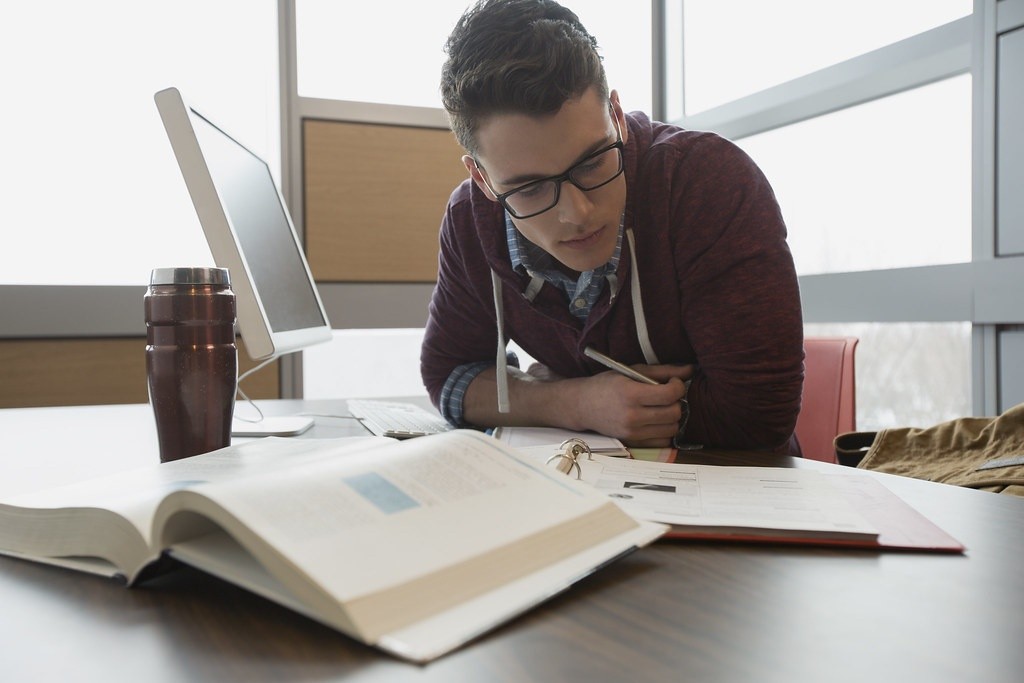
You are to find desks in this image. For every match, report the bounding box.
[0,395,1024,681]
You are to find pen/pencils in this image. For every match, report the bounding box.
[583,346,660,386]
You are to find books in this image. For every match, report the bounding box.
[0,428,670,663]
[494,428,636,463]
[514,439,963,552]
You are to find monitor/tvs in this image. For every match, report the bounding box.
[153,85,334,438]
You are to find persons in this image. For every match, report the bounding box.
[421,0,805,456]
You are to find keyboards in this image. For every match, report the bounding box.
[346,399,452,438]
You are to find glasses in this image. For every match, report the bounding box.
[472,98,626,219]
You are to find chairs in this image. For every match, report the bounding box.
[794,335,859,466]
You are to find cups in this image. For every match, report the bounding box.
[144,267,238,461]
